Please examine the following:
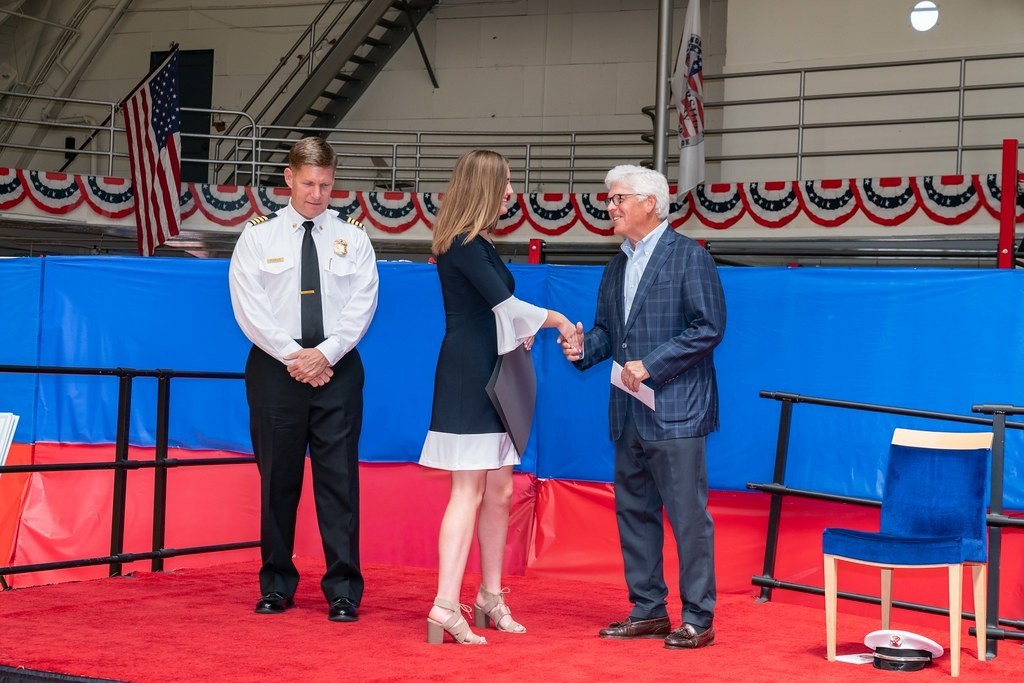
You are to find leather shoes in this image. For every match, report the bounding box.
[663,622,715,650]
[252,590,295,615]
[598,615,671,639]
[327,597,358,622]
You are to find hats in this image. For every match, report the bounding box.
[863,628,945,671]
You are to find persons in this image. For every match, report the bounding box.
[418,149,582,645]
[556,163,727,649]
[227,134,380,621]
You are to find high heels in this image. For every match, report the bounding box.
[473,584,527,634]
[426,596,489,645]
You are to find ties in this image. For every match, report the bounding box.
[300,220,324,348]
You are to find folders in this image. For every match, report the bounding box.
[484,342,537,458]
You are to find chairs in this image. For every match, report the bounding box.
[821,427,995,677]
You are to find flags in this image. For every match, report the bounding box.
[670,0,706,207]
[123,49,181,257]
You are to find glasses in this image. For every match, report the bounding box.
[604,193,649,207]
